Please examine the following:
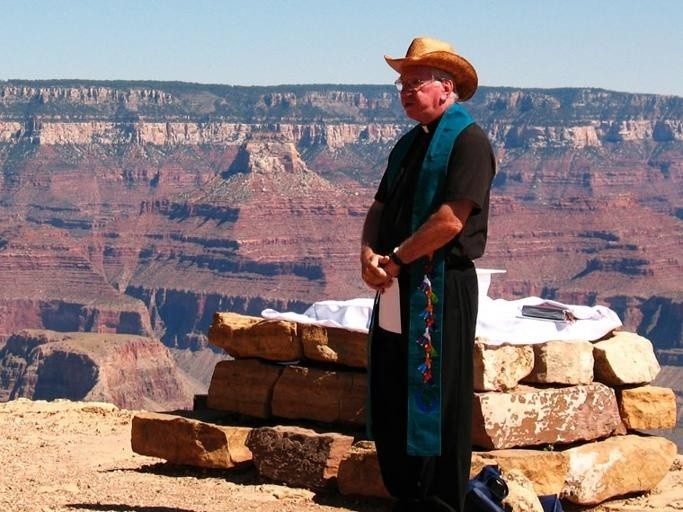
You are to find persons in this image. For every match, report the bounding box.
[359,36,497,511]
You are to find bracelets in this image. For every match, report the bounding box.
[388,246,405,266]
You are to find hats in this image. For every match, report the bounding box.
[383,38,477,103]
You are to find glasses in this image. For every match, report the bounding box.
[394,77,439,92]
